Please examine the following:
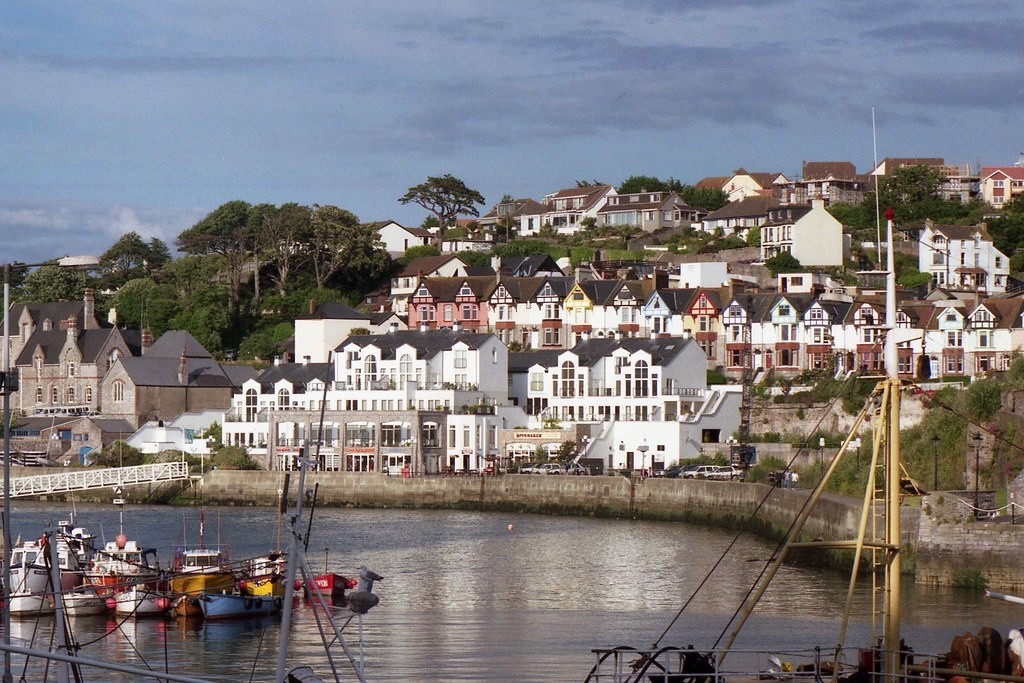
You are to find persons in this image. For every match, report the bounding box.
[739,469,746,482]
[768,467,799,490]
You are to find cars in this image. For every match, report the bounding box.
[519,461,587,476]
[664,465,743,483]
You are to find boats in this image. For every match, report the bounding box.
[0,469,358,623]
